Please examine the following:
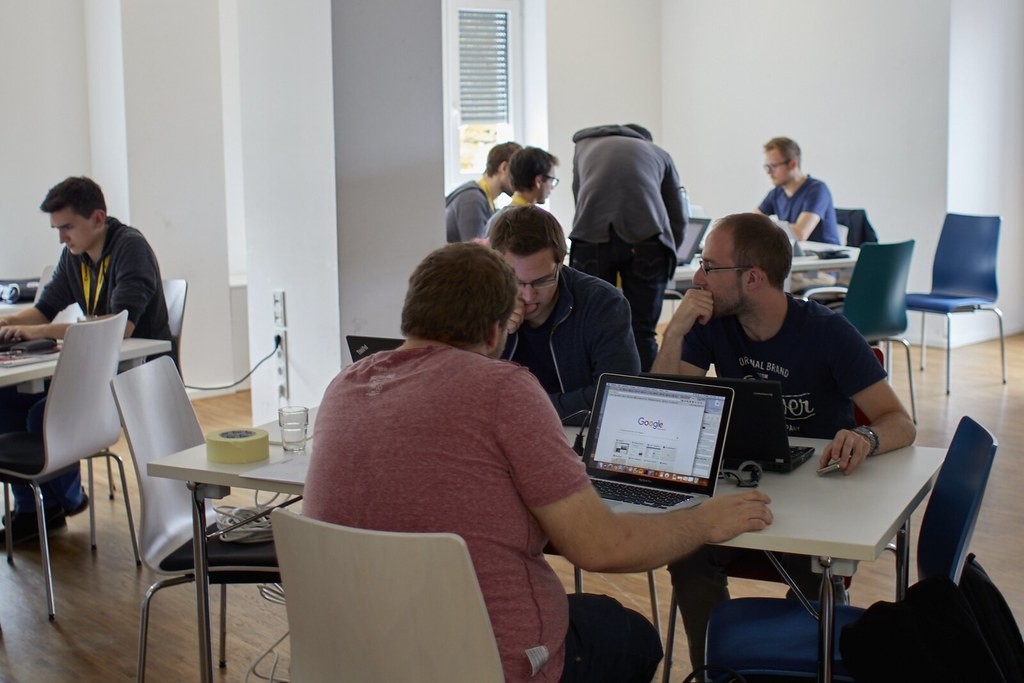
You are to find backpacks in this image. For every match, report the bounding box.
[840,553,1024,683]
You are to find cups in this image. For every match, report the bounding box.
[278,405,309,455]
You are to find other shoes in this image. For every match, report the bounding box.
[0,514,67,550]
[1,485,88,527]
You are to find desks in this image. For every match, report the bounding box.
[106,279,189,499]
[147,408,950,683]
[667,241,863,293]
[0,339,173,388]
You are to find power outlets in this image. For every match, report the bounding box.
[272,332,288,400]
[273,291,286,326]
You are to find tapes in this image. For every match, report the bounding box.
[206,427,269,463]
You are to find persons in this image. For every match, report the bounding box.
[0,176,173,540]
[752,137,840,292]
[650,212,917,683]
[301,241,774,683]
[445,142,561,247]
[484,205,641,427]
[569,123,690,372]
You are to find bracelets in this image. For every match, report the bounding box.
[853,425,879,457]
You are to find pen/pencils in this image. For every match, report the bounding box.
[0,351,24,356]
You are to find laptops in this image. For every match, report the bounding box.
[673,217,712,265]
[581,371,815,513]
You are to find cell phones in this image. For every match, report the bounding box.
[817,458,852,476]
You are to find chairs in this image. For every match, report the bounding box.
[271,507,505,683]
[833,239,918,426]
[34,265,87,345]
[110,355,282,683]
[836,209,877,248]
[706,417,998,683]
[907,213,1009,396]
[662,346,896,683]
[7,309,142,621]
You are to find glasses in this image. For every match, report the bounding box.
[516,260,559,292]
[763,159,792,172]
[534,173,560,189]
[697,260,764,277]
